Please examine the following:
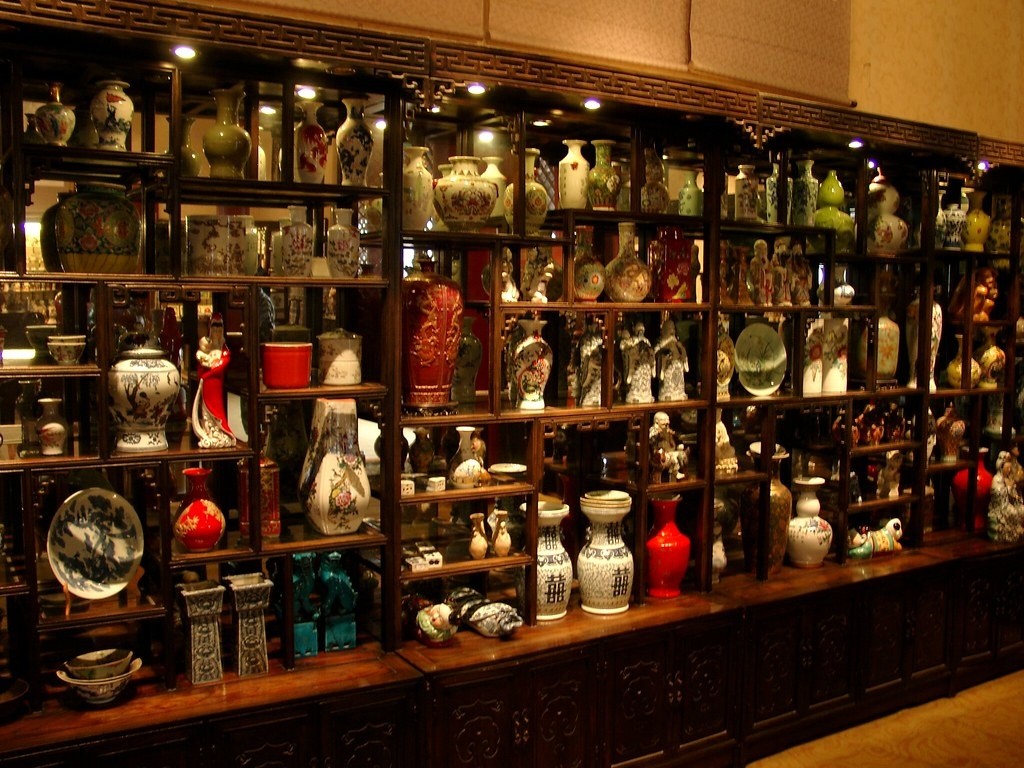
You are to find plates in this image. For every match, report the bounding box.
[733,323,786,396]
[47,488,145,600]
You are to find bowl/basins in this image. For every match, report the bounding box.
[47,336,87,365]
[55,650,142,702]
[26,325,58,354]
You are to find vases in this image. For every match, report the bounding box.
[513,316,554,410]
[605,222,652,301]
[203,87,254,180]
[172,468,222,555]
[557,139,588,210]
[741,442,793,575]
[399,144,433,226]
[334,97,374,186]
[573,224,604,300]
[947,328,982,387]
[590,139,617,211]
[792,158,818,226]
[645,491,689,602]
[293,100,328,180]
[435,155,497,234]
[979,326,1009,389]
[505,146,549,238]
[89,80,136,153]
[788,477,836,564]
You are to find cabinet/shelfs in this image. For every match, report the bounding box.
[0,0,1024,768]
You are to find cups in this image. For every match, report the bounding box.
[321,329,363,386]
[262,344,310,389]
